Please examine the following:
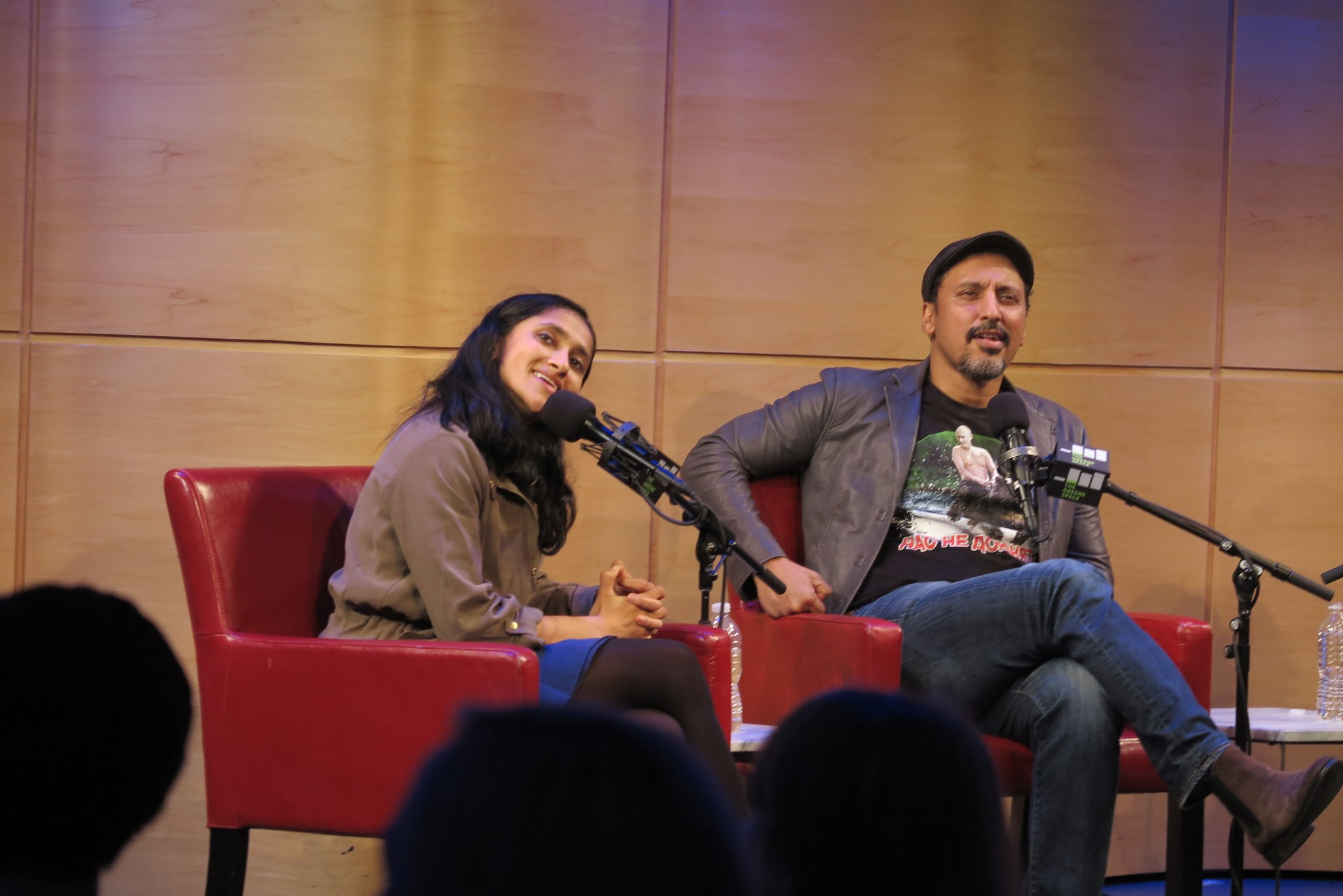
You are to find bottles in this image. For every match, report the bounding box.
[709,602,742,732]
[1317,602,1343,722]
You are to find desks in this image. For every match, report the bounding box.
[1208,707,1343,896]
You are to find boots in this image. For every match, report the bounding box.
[1208,743,1343,869]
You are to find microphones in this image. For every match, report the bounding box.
[539,388,736,551]
[987,392,1040,539]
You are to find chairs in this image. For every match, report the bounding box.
[164,467,735,896]
[720,478,1213,896]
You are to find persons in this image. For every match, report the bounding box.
[679,231,1343,896]
[948,426,999,528]
[0,582,192,896]
[385,700,754,896]
[316,294,747,815]
[747,691,1006,896]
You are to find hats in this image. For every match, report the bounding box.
[922,231,1034,302]
